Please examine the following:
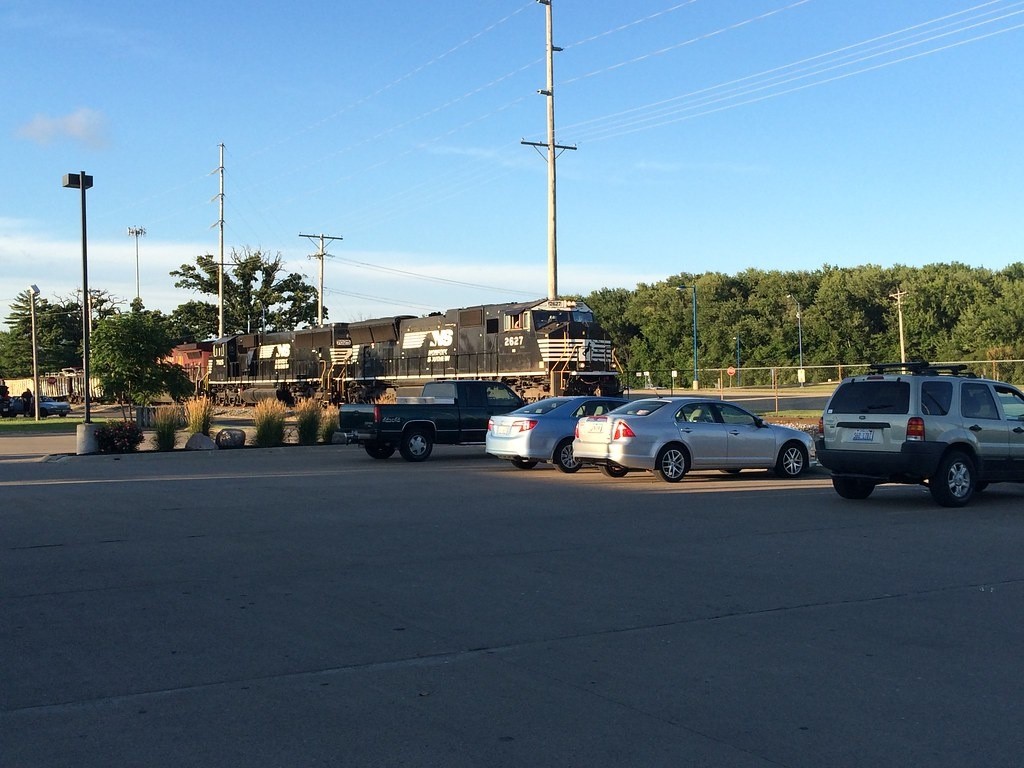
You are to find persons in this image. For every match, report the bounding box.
[22,389,32,417]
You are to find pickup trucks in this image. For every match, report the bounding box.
[331,379,525,460]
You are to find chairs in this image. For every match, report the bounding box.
[594,405,603,416]
[577,406,585,416]
[689,409,705,422]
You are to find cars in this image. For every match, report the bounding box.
[485,395,636,474]
[572,395,817,484]
[0,396,26,417]
[29,396,72,418]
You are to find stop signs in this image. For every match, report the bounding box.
[47,376,57,384]
[727,367,735,376]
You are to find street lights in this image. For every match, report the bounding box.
[786,293,806,389]
[732,328,740,388]
[126,225,146,305]
[675,284,700,382]
[62,171,98,425]
[29,286,41,420]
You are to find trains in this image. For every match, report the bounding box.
[198,298,626,410]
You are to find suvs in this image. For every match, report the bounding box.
[815,361,1024,508]
